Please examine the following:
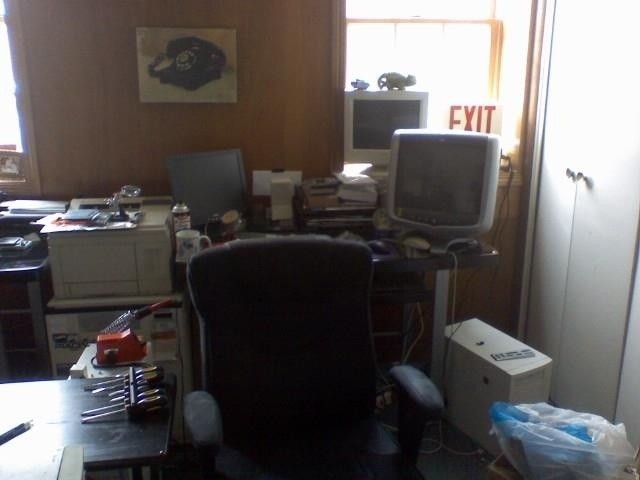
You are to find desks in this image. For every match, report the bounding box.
[0,371,178,479]
[170,219,500,447]
[0,245,59,382]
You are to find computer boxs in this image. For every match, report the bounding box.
[443,317,552,456]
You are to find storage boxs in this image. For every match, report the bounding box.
[41,192,177,300]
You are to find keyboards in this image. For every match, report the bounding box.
[236,231,332,242]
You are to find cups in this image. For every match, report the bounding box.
[174,229,214,262]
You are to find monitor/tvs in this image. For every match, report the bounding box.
[386,128,501,253]
[343,91,428,177]
[166,148,250,238]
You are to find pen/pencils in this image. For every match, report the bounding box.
[0,419,34,446]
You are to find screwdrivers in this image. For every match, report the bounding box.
[80,365,170,423]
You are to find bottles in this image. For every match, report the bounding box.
[171,200,192,252]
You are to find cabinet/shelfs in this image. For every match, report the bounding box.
[40,288,198,454]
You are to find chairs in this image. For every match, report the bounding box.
[174,234,448,479]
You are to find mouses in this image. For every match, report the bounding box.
[366,239,390,255]
